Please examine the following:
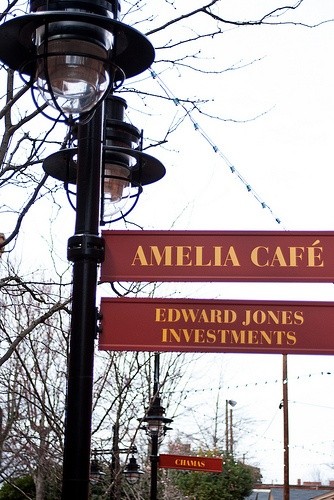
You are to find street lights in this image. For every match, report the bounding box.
[89,436,144,500]
[136,353,174,500]
[0,0,168,500]
[224,399,238,461]
